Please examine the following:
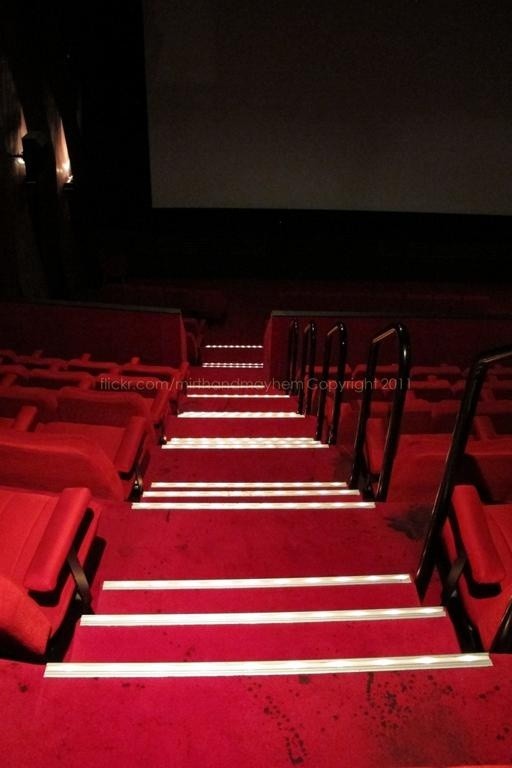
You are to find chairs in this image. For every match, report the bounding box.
[0,281,241,659]
[254,279,512,651]
